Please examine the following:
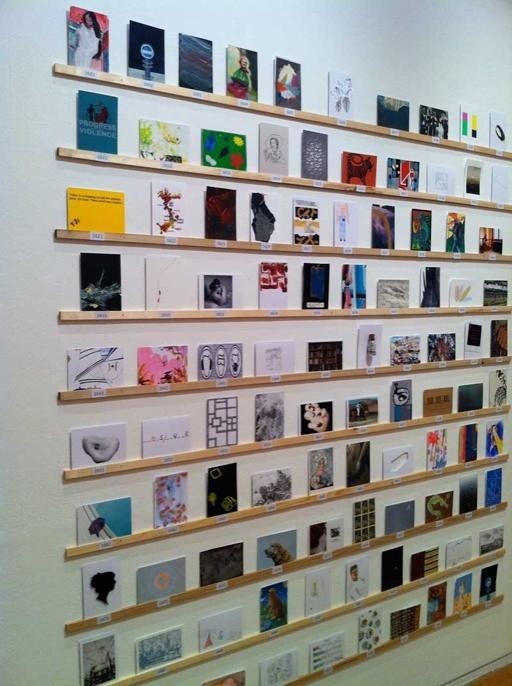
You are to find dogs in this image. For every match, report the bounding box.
[263,541,294,566]
[263,587,284,620]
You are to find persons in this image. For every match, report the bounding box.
[350,564,369,601]
[263,137,286,165]
[228,56,252,98]
[312,457,332,489]
[74,11,102,68]
